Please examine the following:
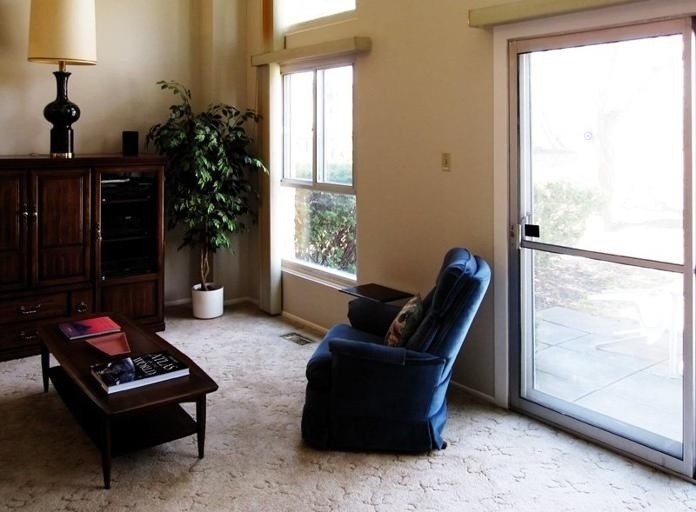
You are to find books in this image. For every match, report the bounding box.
[59,316,121,339]
[90,349,190,394]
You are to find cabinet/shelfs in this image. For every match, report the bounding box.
[0,155,165,360]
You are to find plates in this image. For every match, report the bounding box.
[85,331,132,358]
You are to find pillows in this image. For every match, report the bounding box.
[383,292,423,348]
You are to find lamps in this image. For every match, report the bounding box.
[27,0,98,158]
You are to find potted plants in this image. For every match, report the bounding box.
[143,79,270,319]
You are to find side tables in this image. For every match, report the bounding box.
[338,283,414,338]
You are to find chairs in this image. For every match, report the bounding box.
[301,246,492,454]
